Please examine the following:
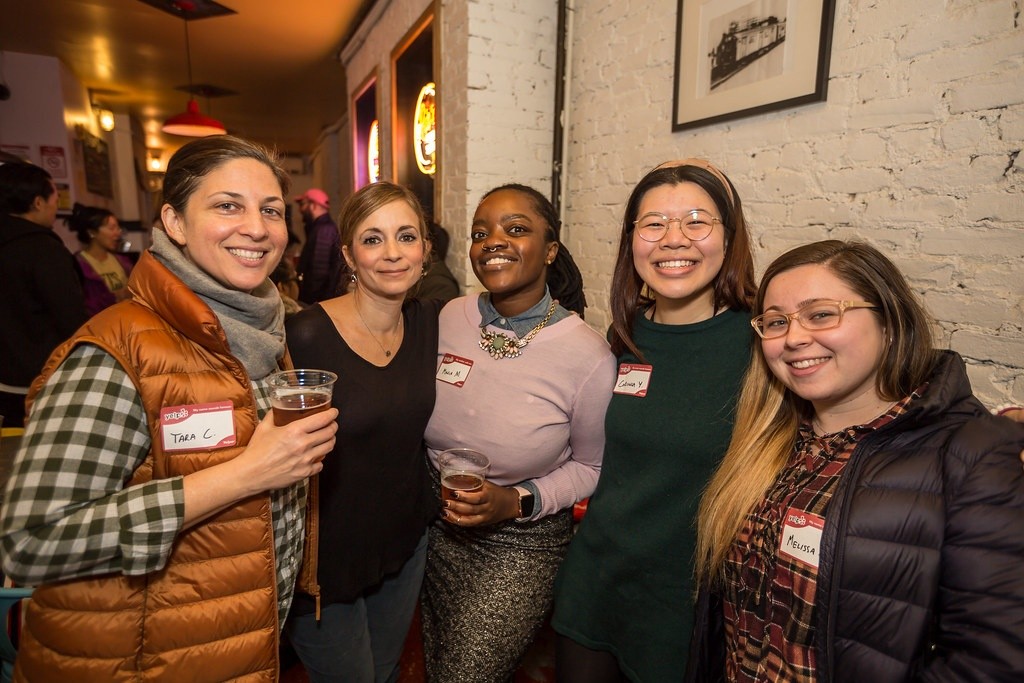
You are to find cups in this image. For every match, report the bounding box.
[439,448,491,515]
[265,369,338,463]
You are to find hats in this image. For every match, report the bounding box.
[294,189,329,209]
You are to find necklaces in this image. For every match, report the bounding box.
[478,300,556,359]
[814,400,894,436]
[354,285,403,357]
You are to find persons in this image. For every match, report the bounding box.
[283,178,438,683]
[547,162,760,683]
[683,240,1024,683]
[0,133,340,683]
[420,182,617,683]
[0,158,462,429]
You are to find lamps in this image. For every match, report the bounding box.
[162,0,226,136]
[99,107,115,132]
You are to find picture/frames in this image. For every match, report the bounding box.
[671,0,837,133]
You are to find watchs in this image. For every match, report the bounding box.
[512,485,536,518]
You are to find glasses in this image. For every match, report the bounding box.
[632,209,723,241]
[751,300,880,339]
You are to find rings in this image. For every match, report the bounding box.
[457,518,460,525]
